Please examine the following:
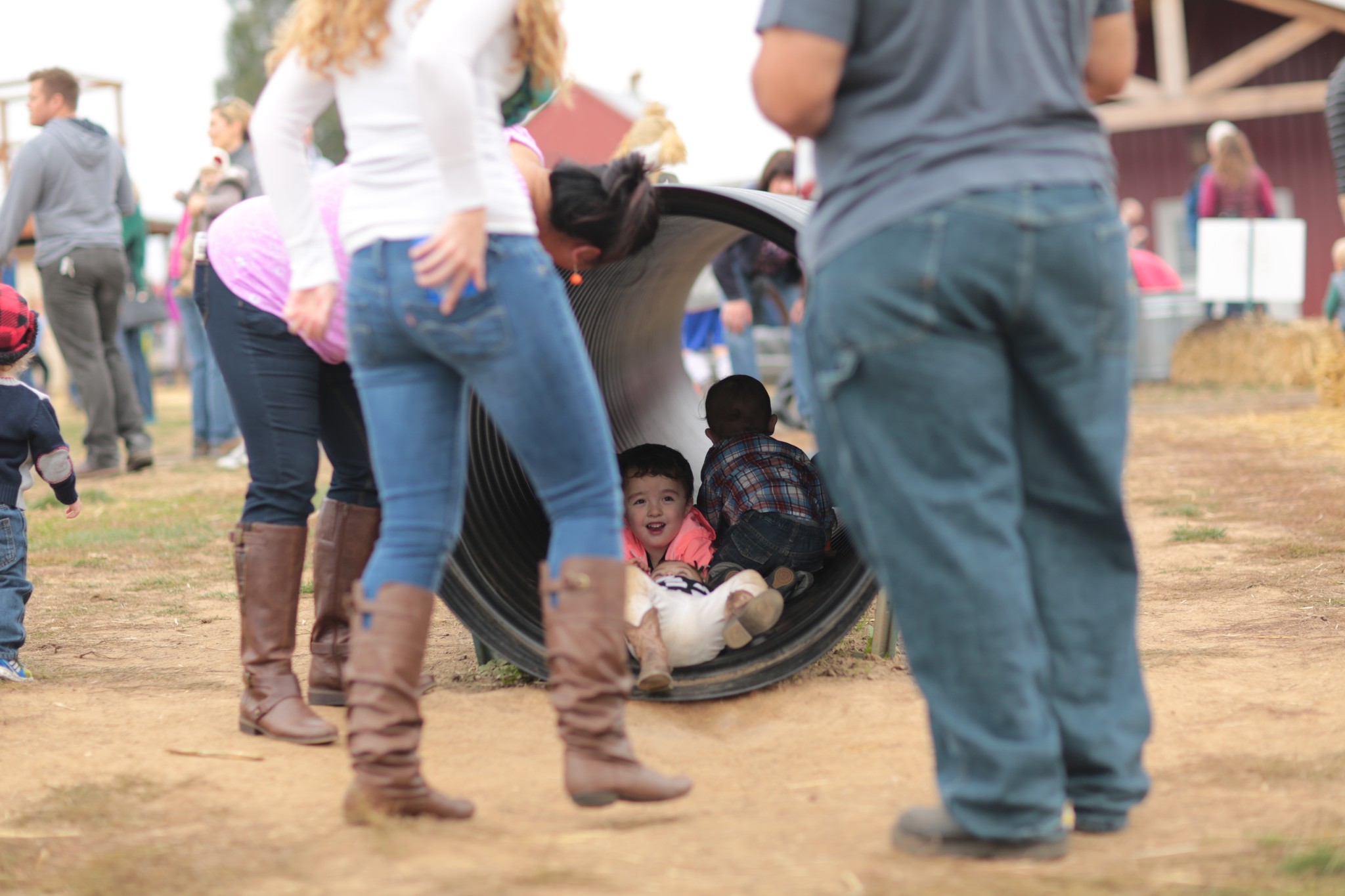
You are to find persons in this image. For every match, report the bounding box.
[712,150,812,426]
[192,125,661,745]
[169,96,262,470]
[0,284,80,682]
[618,443,716,577]
[1325,56,1345,220]
[752,0,1155,866]
[623,559,783,692]
[120,182,155,423]
[0,68,155,476]
[1324,236,1345,331]
[697,375,838,602]
[1119,197,1148,248]
[248,0,695,826]
[1184,121,1273,317]
[681,263,733,384]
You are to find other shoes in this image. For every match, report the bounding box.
[896,807,1063,862]
[76,463,120,478]
[216,446,248,468]
[127,450,153,469]
[766,566,797,594]
[1076,809,1122,832]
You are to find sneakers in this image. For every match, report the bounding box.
[0,658,33,681]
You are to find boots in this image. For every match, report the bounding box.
[307,496,380,706]
[344,581,473,824]
[229,521,338,744]
[540,556,692,805]
[622,605,673,693]
[722,589,782,650]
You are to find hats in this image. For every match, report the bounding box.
[0,281,38,363]
